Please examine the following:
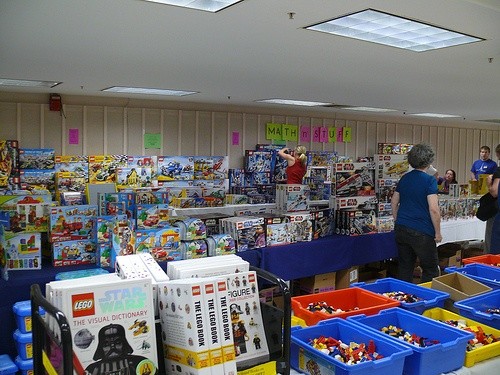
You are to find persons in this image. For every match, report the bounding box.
[476,144,500,255]
[390,143,442,283]
[431,167,444,184]
[278,145,307,184]
[469,145,497,180]
[440,168,457,193]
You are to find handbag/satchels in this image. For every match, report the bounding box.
[476,192,498,221]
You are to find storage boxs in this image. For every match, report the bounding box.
[0,354,19,375]
[13,328,33,359]
[13,300,46,334]
[0,140,492,269]
[258,243,500,375]
[15,355,35,375]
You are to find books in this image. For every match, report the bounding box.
[45,251,269,375]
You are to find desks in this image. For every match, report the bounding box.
[0,231,397,361]
[169,199,329,224]
[437,216,487,276]
